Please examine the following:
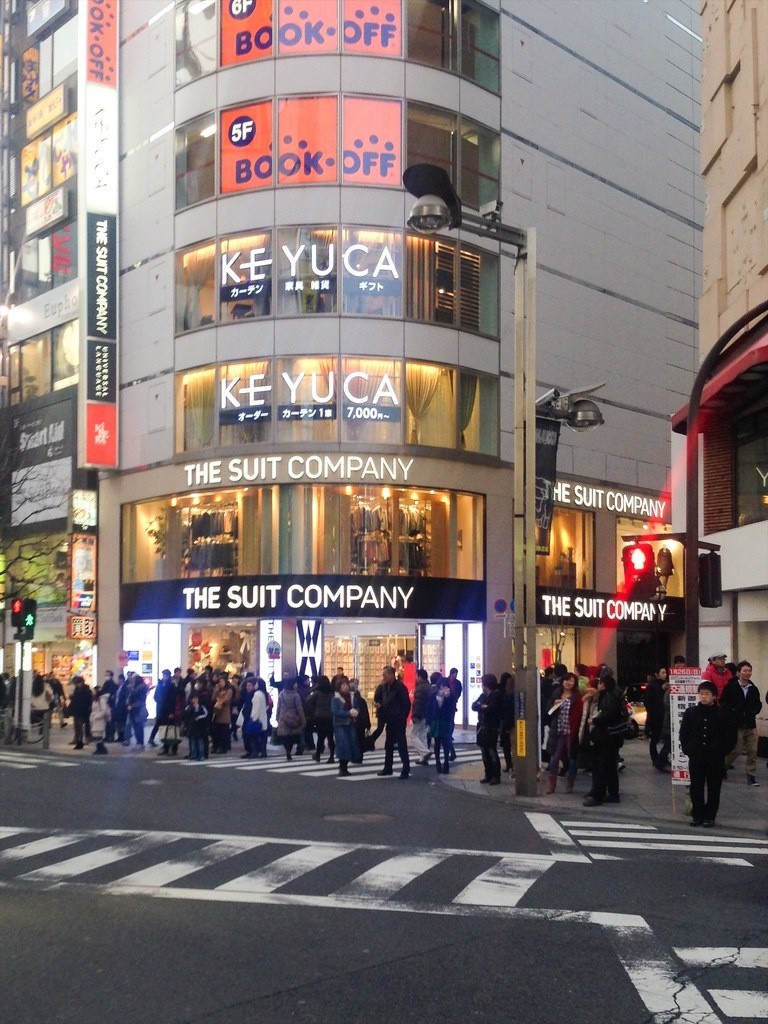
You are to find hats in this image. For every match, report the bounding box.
[710,653,727,662]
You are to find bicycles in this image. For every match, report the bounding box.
[0,699,45,744]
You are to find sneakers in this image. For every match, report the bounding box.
[747,776,759,786]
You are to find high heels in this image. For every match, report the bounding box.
[504,761,513,772]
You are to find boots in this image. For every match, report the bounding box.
[564,774,574,793]
[546,774,556,794]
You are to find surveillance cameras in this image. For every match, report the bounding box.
[535,388,560,407]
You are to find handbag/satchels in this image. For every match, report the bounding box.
[159,726,180,740]
[284,710,300,729]
[624,719,639,739]
[62,702,70,719]
[245,720,262,735]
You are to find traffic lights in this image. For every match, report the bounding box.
[12,597,37,640]
[622,543,657,600]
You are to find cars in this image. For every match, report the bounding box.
[621,681,652,736]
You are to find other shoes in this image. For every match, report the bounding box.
[617,762,625,773]
[450,754,457,761]
[68,732,410,779]
[436,762,449,773]
[479,776,501,785]
[689,818,716,827]
[728,764,734,770]
[583,795,621,806]
[415,752,433,766]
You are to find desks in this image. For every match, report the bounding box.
[230,304,252,319]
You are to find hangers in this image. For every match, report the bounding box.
[192,502,422,545]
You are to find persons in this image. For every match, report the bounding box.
[472,663,631,807]
[147,659,462,779]
[680,681,738,828]
[66,669,149,754]
[640,652,768,786]
[0,672,67,733]
